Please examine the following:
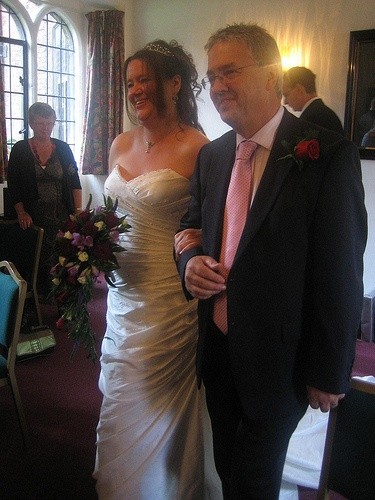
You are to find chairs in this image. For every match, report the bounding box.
[0,219,45,326]
[316,378,375,500]
[0,260,33,449]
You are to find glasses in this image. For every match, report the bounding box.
[33,121,54,127]
[201,63,262,90]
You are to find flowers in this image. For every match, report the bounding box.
[277,137,320,168]
[48,190,132,370]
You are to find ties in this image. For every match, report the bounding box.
[211,140,259,336]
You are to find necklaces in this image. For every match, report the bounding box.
[144,124,178,153]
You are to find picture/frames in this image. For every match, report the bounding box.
[343,28,375,160]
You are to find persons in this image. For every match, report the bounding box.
[93,39,211,500]
[7,102,83,321]
[172,24,367,500]
[282,68,344,137]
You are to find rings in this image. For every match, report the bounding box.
[331,403,336,406]
[21,222,23,223]
